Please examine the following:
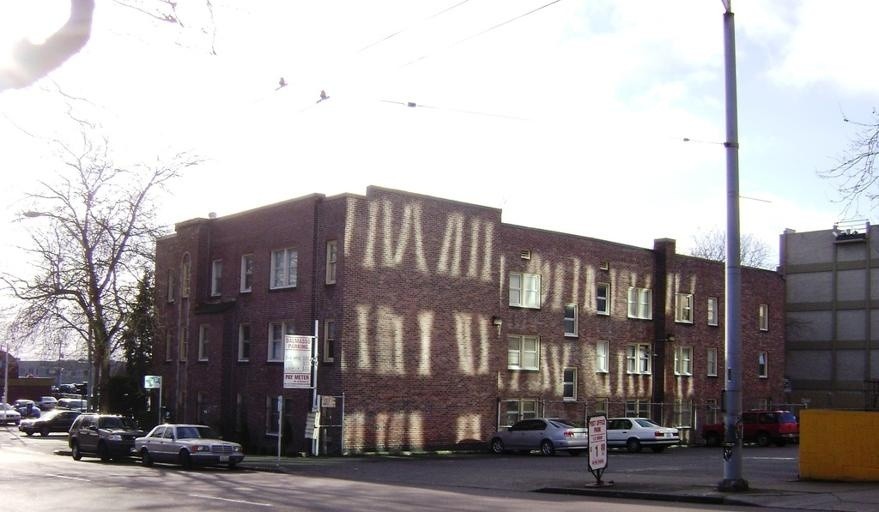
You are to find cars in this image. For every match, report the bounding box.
[19,409,82,437]
[486,417,588,456]
[0,395,58,424]
[135,423,244,467]
[607,417,679,451]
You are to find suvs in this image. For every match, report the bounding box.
[68,413,145,461]
[704,410,799,446]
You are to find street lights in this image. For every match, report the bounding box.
[26,209,94,410]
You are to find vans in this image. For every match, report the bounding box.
[56,398,88,412]
